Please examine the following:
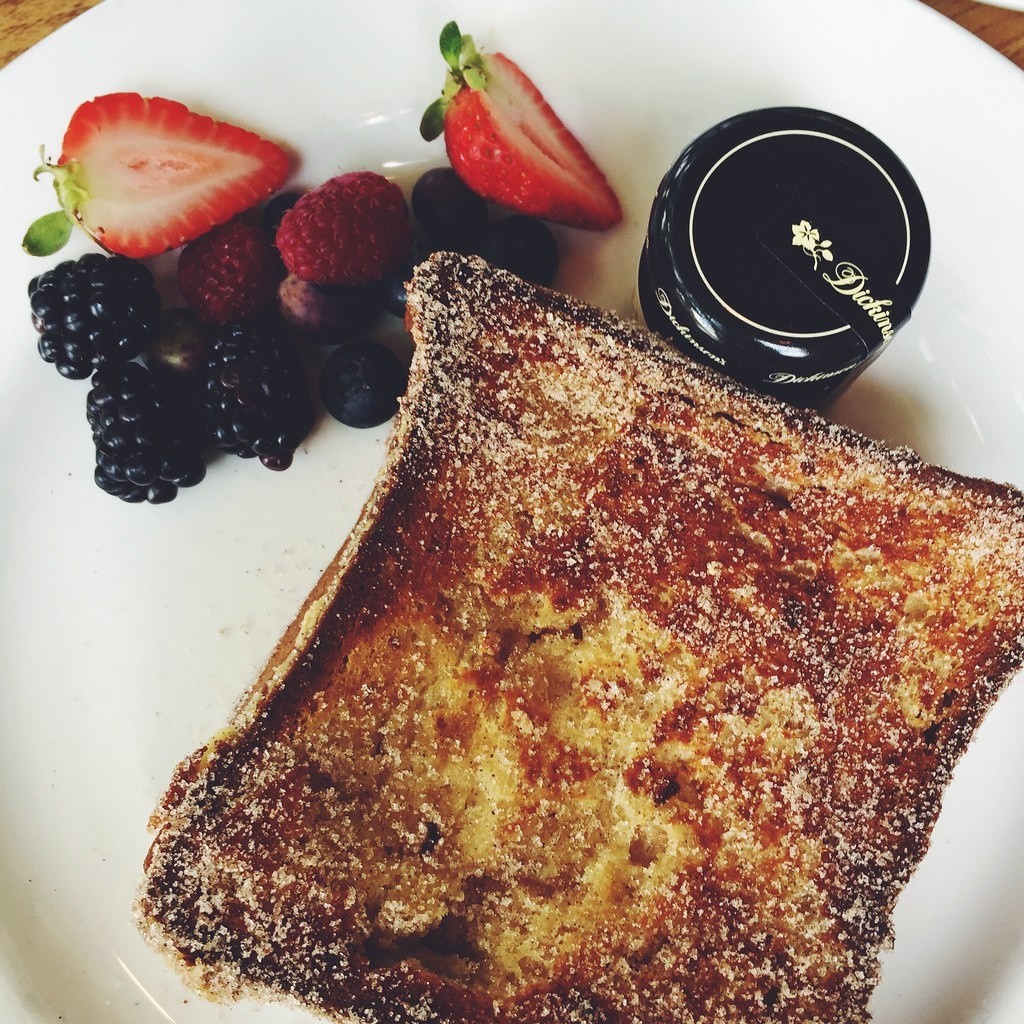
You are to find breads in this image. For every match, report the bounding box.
[132,249,1024,1023]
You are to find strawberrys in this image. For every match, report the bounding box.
[421,22,624,234]
[21,90,292,259]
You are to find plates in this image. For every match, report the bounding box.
[0,0,1024,1024]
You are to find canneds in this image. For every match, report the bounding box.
[638,105,930,417]
